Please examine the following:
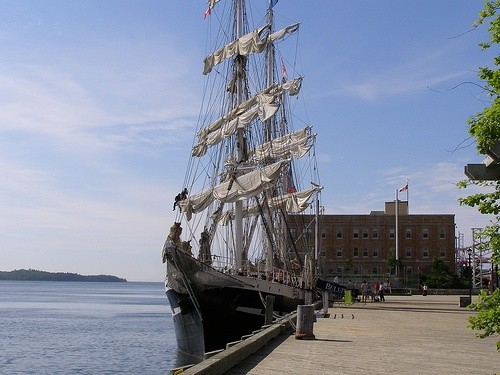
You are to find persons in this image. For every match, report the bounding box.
[360,280,385,302]
[347,280,354,288]
[421,283,428,296]
[386,279,391,293]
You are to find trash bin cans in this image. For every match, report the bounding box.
[459,297,469,308]
[296,305,314,339]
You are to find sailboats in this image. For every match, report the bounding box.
[161,1,325,360]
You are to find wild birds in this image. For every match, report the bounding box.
[333,313,337,319]
[351,314,354,319]
[341,313,344,318]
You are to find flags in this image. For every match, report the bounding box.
[204,6,211,19]
[399,184,408,192]
[282,64,287,76]
